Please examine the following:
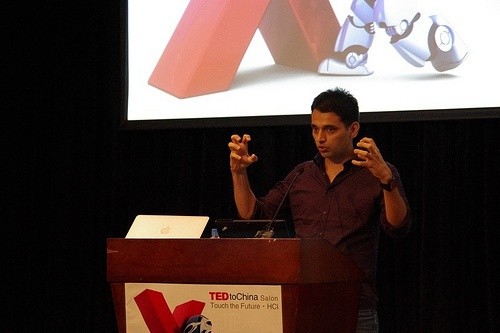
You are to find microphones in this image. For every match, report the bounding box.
[267,168,304,231]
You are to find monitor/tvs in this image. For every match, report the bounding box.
[214,218,291,239]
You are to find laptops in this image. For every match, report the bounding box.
[124,214,209,239]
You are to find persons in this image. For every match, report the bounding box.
[228,86,409,332]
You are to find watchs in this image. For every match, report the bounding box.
[380,177,398,192]
